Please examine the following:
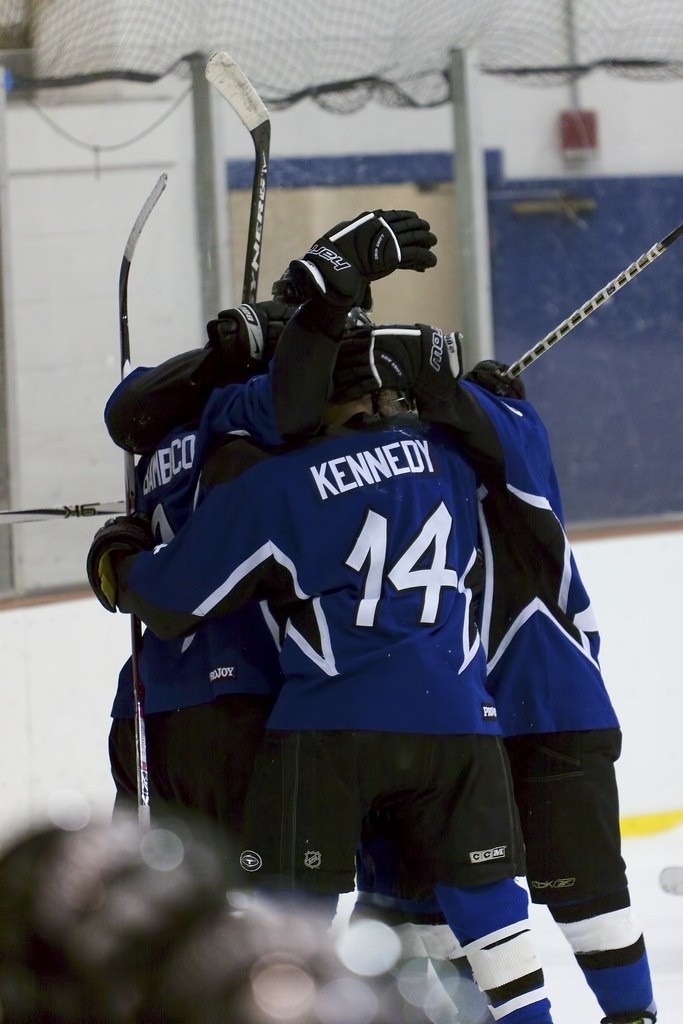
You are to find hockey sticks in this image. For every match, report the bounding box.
[501,224,683,387]
[0,501,148,526]
[204,51,272,303]
[119,172,167,828]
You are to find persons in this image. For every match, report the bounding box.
[0,210,658,1024]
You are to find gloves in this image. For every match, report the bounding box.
[290,210,436,312]
[86,515,154,613]
[466,359,525,400]
[208,303,299,376]
[331,324,462,402]
